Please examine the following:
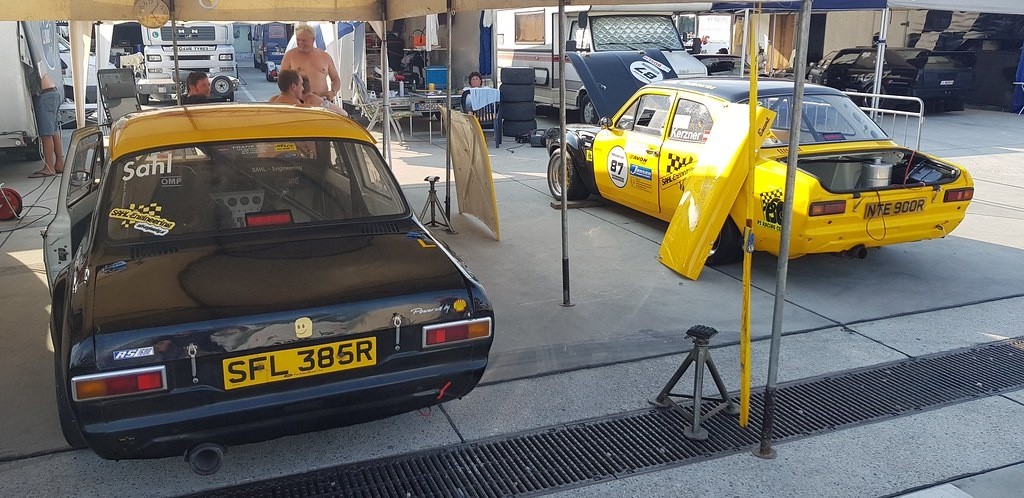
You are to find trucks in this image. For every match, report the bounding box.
[279,8,498,121]
[0,19,238,161]
[494,2,713,125]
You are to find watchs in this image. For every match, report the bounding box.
[331,89,337,96]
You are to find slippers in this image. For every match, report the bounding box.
[29,171,55,178]
[56,171,63,174]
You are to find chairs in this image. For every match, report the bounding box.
[96,68,141,127]
[461,87,503,148]
[348,72,404,146]
[137,163,233,238]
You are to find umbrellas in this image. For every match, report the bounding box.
[311,21,363,51]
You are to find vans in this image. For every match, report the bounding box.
[247,22,291,73]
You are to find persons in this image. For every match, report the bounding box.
[181,72,211,105]
[458,71,489,116]
[269,25,348,118]
[27,75,64,178]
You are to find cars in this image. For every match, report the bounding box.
[693,53,752,77]
[772,46,973,117]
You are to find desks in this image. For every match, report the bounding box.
[408,92,462,144]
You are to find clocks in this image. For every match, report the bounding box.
[134,0,169,29]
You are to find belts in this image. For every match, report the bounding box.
[41,87,57,94]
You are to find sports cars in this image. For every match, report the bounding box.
[39,100,497,476]
[545,48,974,282]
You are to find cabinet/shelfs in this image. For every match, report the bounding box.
[365,32,381,68]
[402,48,447,91]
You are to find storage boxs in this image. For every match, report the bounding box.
[425,66,447,90]
